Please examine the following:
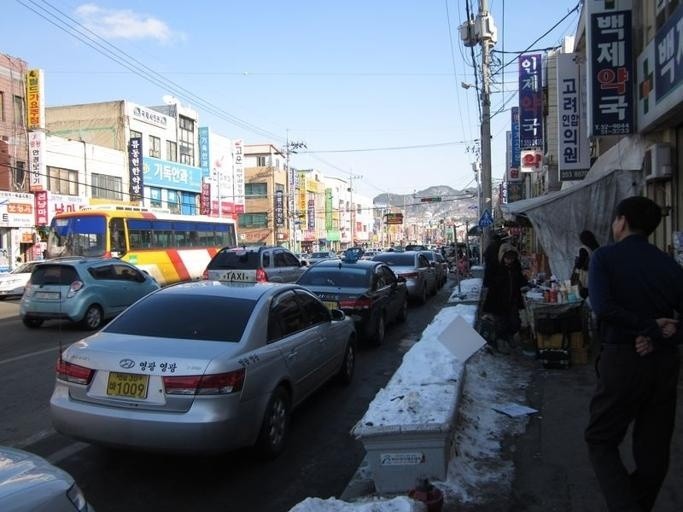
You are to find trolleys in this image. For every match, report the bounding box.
[519,284,591,366]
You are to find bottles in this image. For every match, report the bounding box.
[532,273,577,304]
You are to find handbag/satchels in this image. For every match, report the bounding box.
[576,268,587,288]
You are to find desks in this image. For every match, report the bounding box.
[522,296,585,361]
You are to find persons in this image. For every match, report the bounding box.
[579,196,683,511]
[574,229,600,306]
[17,257,23,267]
[0,249,9,266]
[437,246,481,268]
[473,233,529,355]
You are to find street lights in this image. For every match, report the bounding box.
[458,80,494,262]
[68,138,87,197]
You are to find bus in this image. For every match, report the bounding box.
[47,198,237,286]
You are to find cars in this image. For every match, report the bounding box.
[0,445,96,512]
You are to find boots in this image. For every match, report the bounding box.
[495,338,511,355]
